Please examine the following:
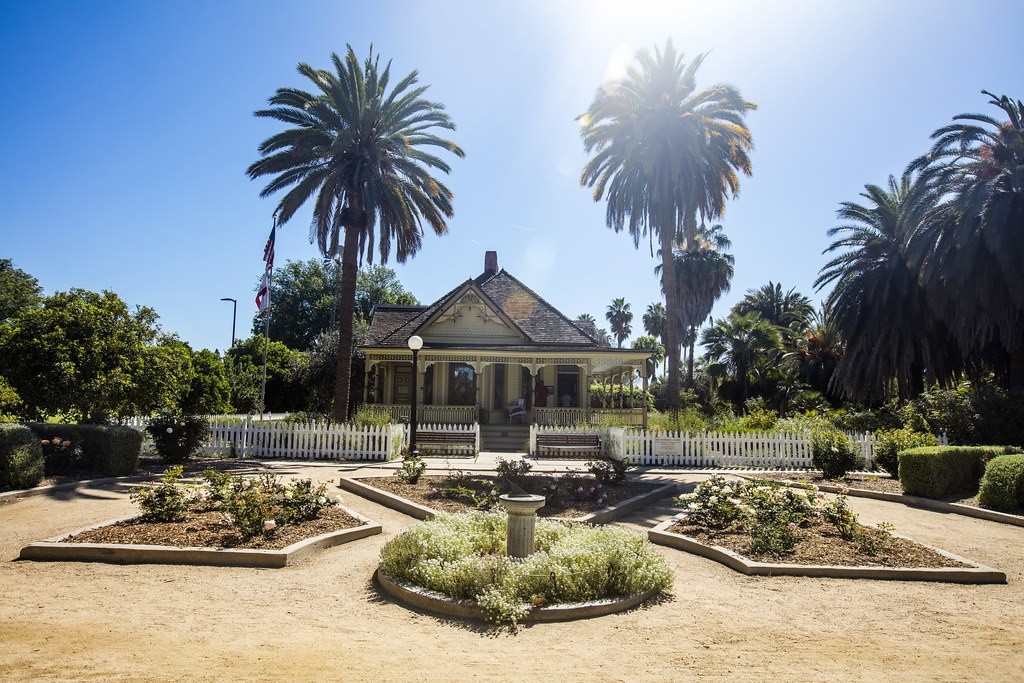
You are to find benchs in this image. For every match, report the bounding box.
[415,431,476,458]
[536,433,602,461]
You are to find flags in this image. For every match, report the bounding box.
[255,270,270,315]
[263,225,275,272]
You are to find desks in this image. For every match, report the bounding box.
[503,404,527,425]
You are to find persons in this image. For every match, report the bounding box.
[560,390,572,408]
[534,380,550,407]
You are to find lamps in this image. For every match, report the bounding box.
[408,335,423,350]
